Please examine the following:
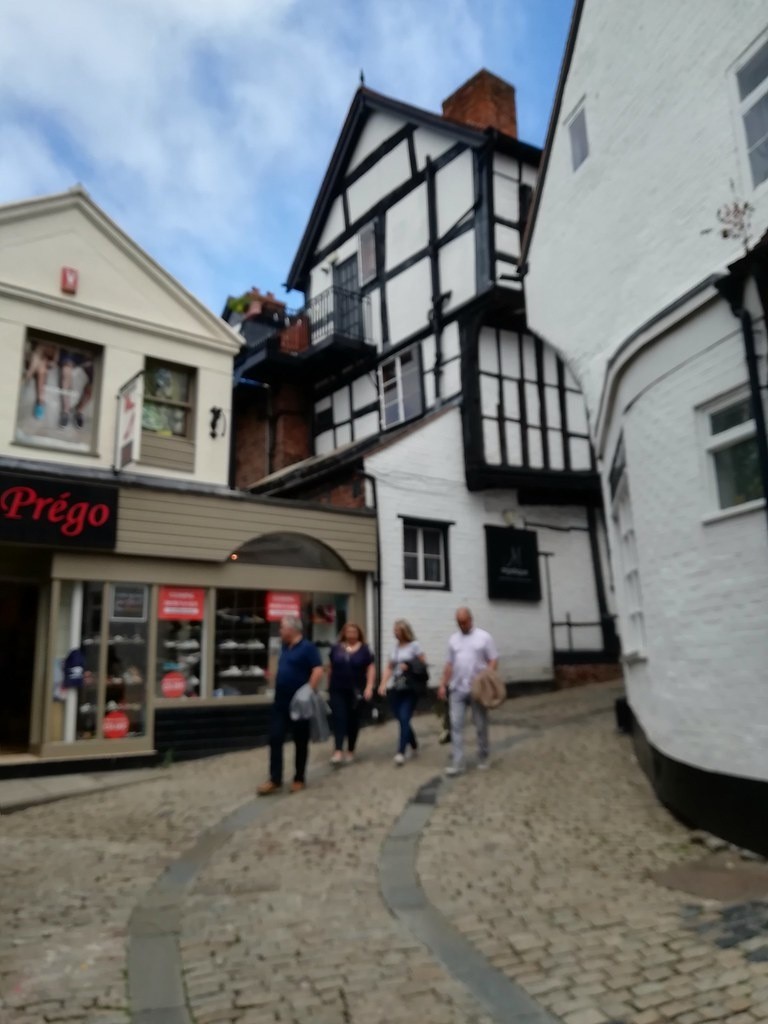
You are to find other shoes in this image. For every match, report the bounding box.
[446,767,465,775]
[256,781,277,794]
[393,749,417,764]
[290,781,304,792]
[478,757,491,770]
[329,752,354,764]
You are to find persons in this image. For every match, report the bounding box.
[438,608,499,777]
[377,619,427,765]
[24,340,94,429]
[321,624,376,763]
[254,617,323,794]
